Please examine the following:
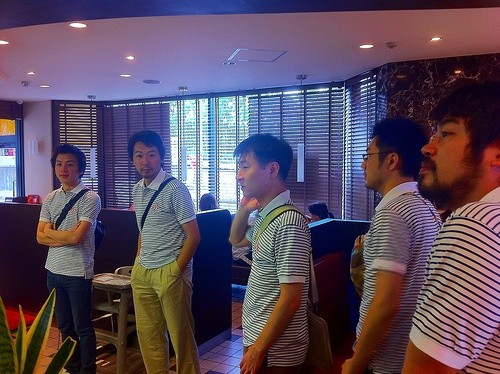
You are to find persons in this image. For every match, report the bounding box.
[232,208,262,267]
[128,130,201,374]
[228,133,312,374]
[341,118,442,374]
[401,84,500,374]
[305,202,335,224]
[199,193,218,211]
[36,142,101,374]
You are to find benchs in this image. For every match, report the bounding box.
[309,253,358,374]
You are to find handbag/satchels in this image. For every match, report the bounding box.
[94,221,105,248]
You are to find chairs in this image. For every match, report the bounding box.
[92,266,143,374]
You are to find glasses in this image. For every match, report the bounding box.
[362,151,389,160]
